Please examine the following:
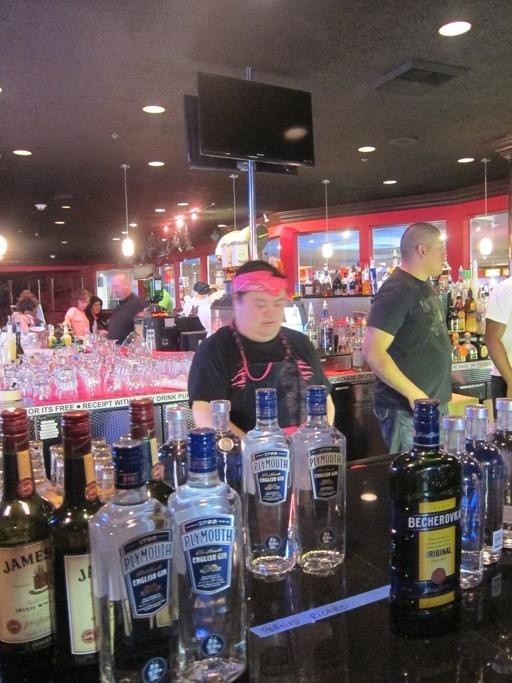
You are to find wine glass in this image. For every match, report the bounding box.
[1,325,191,397]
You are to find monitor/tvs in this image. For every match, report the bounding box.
[184,94,299,175]
[196,70,315,167]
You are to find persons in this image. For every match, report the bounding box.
[363,222,452,456]
[0,271,174,357]
[186,282,210,317]
[188,260,336,441]
[484,276,512,417]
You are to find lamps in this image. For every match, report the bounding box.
[121,164,135,256]
[322,180,333,259]
[479,158,492,254]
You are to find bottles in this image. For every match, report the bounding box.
[127,398,175,506]
[298,301,367,371]
[439,258,491,363]
[2,406,57,683]
[47,410,115,683]
[157,407,189,491]
[241,385,298,585]
[386,400,512,636]
[309,248,399,294]
[166,427,248,683]
[295,385,355,577]
[86,438,180,683]
[208,400,242,495]
